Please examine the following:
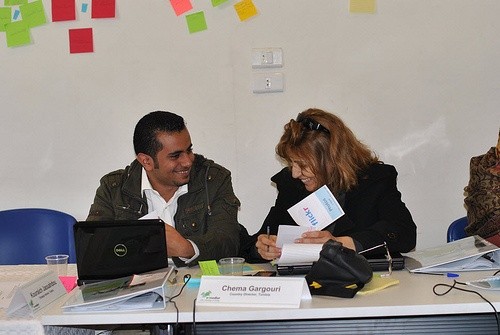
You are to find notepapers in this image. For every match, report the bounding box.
[358,271,399,294]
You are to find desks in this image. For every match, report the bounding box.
[1,264,500,335]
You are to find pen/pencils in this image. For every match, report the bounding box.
[267,226,270,253]
[414,271,460,278]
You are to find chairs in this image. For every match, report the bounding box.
[0,208,78,265]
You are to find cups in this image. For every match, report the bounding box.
[219,257,245,276]
[45,255,69,278]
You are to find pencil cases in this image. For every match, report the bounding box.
[304,239,374,298]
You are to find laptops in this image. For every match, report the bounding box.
[276,248,405,275]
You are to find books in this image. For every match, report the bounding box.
[401,235,500,272]
[60,267,178,311]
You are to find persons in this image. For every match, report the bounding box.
[243,108,417,264]
[85,110,241,267]
[463,129,500,238]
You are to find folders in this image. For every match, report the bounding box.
[60,263,179,312]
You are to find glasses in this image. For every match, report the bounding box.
[297,113,331,138]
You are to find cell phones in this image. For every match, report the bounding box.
[254,271,277,276]
